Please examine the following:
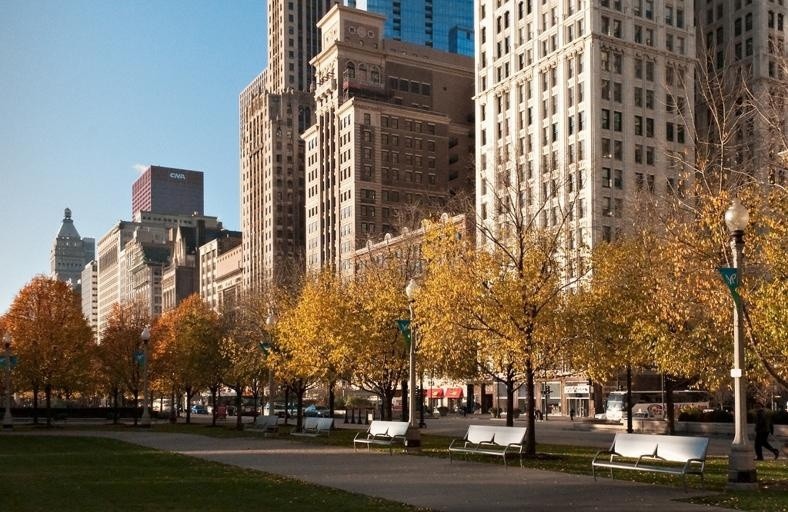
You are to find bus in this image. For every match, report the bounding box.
[206,394,266,416]
[605,390,725,425]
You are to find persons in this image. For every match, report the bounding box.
[753,397,780,461]
[570,408,575,420]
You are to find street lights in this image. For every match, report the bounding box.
[1,331,13,418]
[265,313,277,416]
[724,197,751,451]
[140,327,150,415]
[406,278,419,429]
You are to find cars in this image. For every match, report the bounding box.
[261,402,331,417]
[190,405,205,415]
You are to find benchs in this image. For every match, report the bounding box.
[243,413,410,458]
[590,431,709,494]
[447,424,527,470]
